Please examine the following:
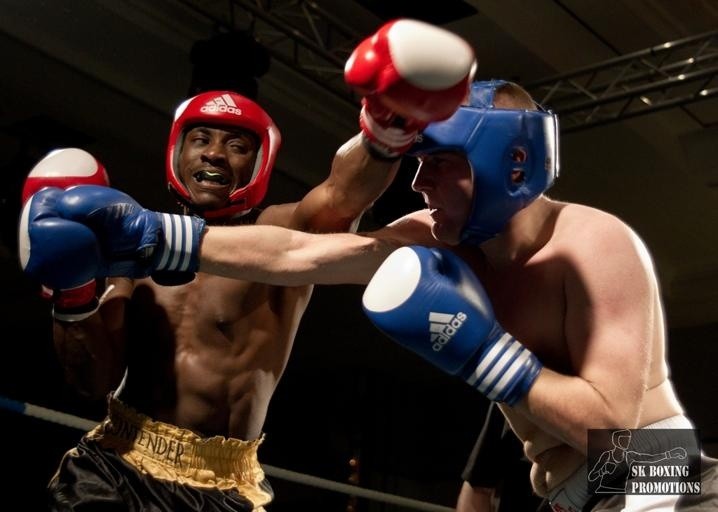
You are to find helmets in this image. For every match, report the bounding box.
[164,91,282,220]
[415,105,560,243]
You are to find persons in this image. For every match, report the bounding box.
[17,75,717,512]
[19,18,480,511]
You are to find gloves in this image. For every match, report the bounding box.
[22,148,112,322]
[361,244,543,408]
[18,185,206,289]
[343,16,477,158]
